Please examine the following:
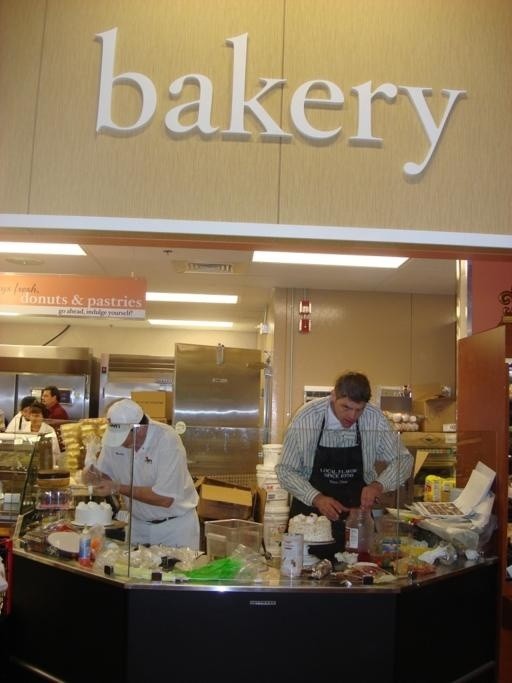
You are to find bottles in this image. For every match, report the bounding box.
[344,507,375,561]
[79,522,91,566]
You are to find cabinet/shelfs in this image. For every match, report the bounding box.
[1,431,54,537]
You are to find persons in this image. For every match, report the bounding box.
[41,384,70,452]
[4,394,38,434]
[274,370,416,561]
[18,401,60,468]
[79,397,203,554]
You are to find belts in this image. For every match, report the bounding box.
[149,516,176,523]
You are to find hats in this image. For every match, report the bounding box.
[102,398,144,447]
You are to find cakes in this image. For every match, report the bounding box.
[74,501,113,524]
[288,513,333,543]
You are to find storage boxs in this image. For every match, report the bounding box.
[205,519,264,562]
[195,476,254,521]
[130,391,174,423]
[417,393,457,432]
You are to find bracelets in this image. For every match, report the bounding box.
[111,479,122,495]
